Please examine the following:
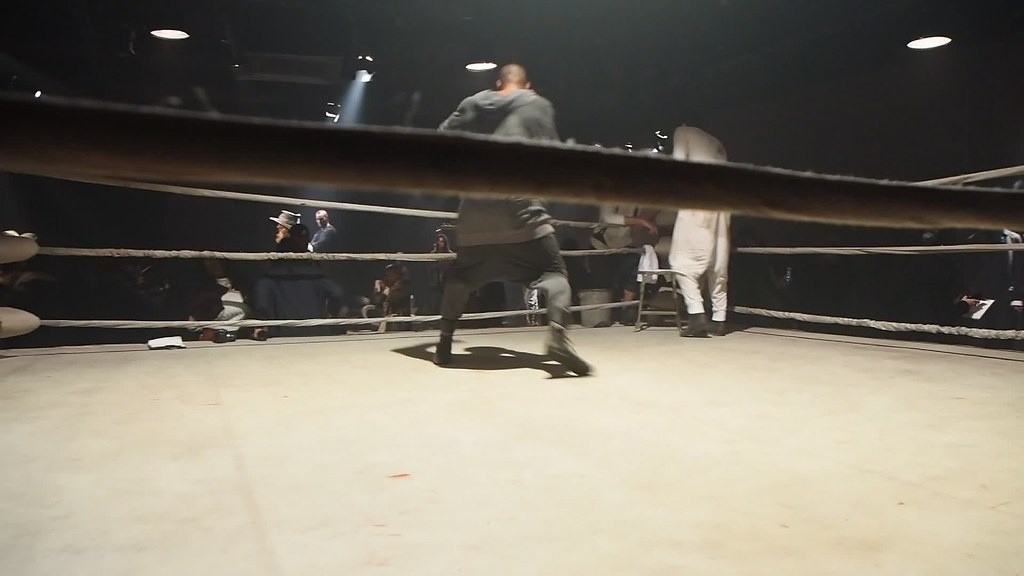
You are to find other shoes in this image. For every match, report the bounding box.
[680,314,709,336]
[433,334,453,365]
[710,321,729,336]
[546,320,591,376]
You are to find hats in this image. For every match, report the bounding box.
[632,223,661,248]
[269,210,301,230]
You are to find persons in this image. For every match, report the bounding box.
[587,199,658,329]
[0,207,415,340]
[428,61,592,378]
[914,228,1024,330]
[667,112,733,338]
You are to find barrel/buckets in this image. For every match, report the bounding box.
[579,288,614,327]
